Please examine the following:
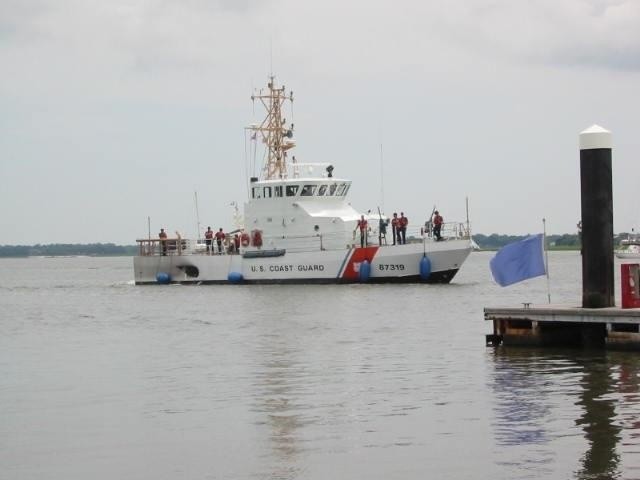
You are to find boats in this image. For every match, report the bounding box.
[614,242,640,259]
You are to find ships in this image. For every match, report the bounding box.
[131,72,475,286]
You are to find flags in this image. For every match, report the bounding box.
[489,232,546,287]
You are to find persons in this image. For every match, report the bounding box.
[380,218,388,246]
[159,229,167,256]
[355,215,368,248]
[205,227,263,255]
[392,213,401,244]
[433,210,443,240]
[397,212,408,244]
